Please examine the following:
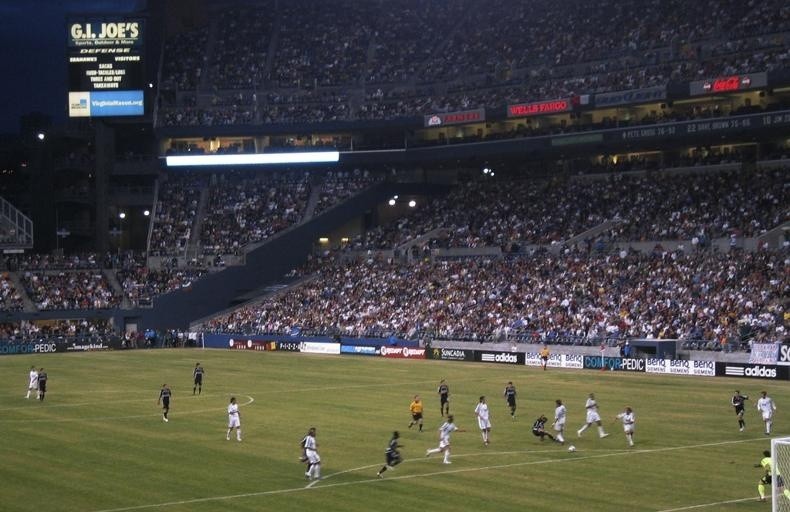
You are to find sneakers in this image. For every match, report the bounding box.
[600,433,609,438]
[444,460,453,465]
[629,441,633,446]
[377,472,383,479]
[226,434,231,440]
[304,472,323,480]
[236,437,242,442]
[756,497,767,502]
[577,430,582,437]
[385,465,394,472]
[24,395,44,401]
[426,449,431,457]
[162,416,169,423]
[739,424,745,432]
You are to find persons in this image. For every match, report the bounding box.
[756,451,790,502]
[300,428,315,479]
[758,392,777,435]
[157,384,171,423]
[377,379,636,478]
[226,398,242,442]
[25,365,40,399]
[192,363,205,395]
[38,368,48,402]
[304,430,321,480]
[732,391,748,432]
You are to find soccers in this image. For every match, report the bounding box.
[569,445,575,452]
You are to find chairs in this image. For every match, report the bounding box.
[196,246,788,359]
[47,254,100,270]
